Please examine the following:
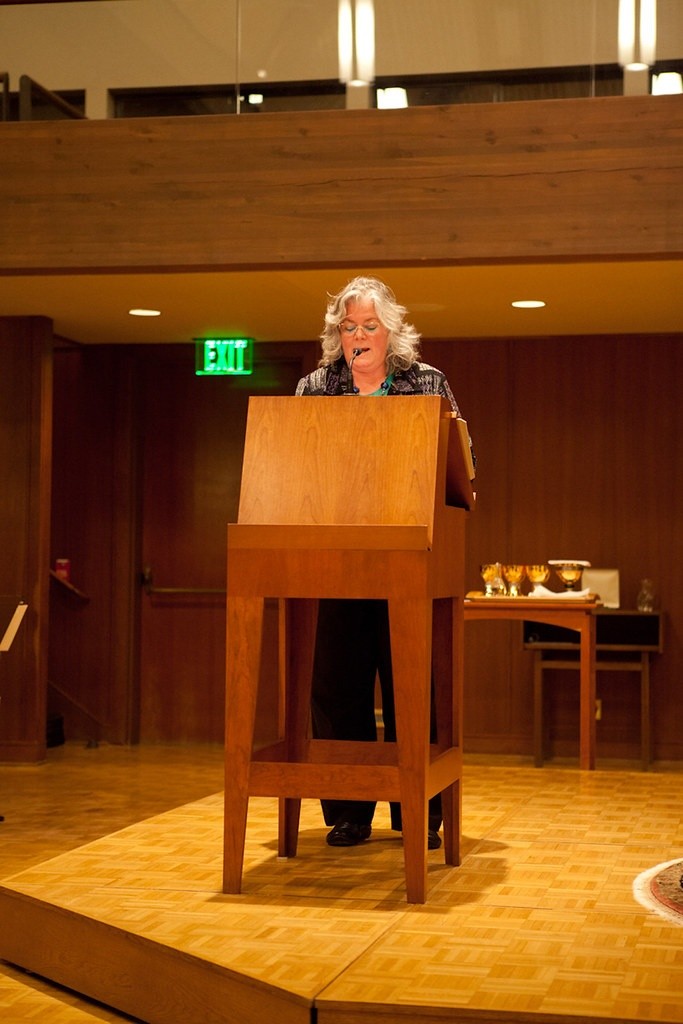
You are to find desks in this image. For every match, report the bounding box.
[464,598,605,772]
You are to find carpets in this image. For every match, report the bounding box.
[633,857,682,928]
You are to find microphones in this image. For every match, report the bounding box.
[347,348,362,393]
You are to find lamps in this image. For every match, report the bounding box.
[336,0,376,87]
[618,0,657,71]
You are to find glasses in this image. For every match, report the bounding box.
[339,321,383,336]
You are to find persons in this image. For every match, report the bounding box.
[293,278,479,851]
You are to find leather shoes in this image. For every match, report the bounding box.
[428,830,441,849]
[326,821,371,846]
